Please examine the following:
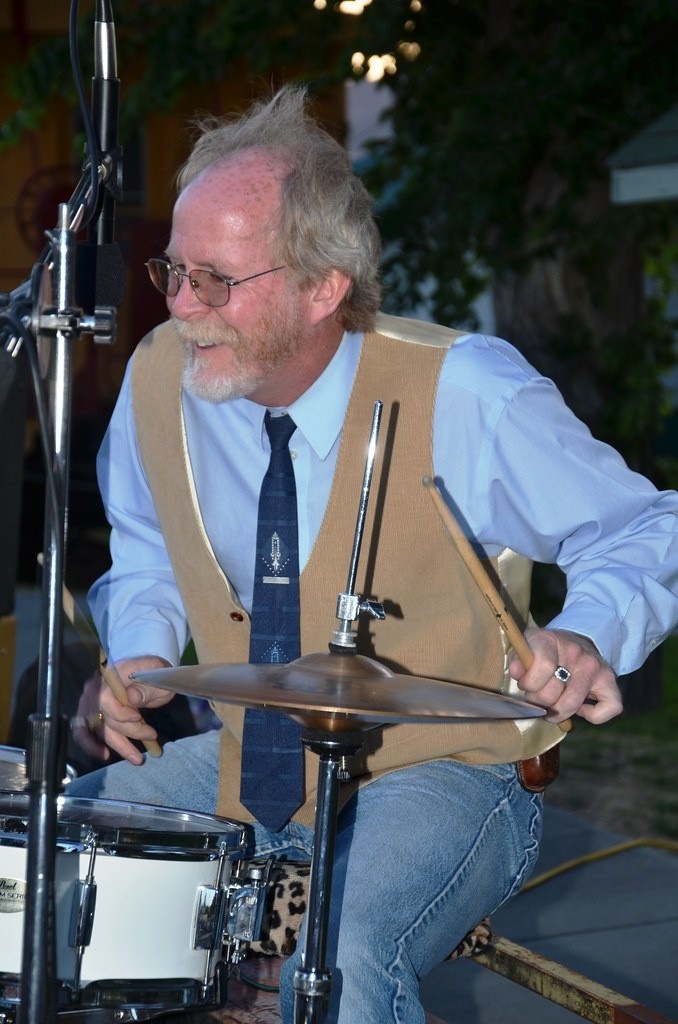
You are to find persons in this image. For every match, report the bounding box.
[67,81,678,1024]
[504,258,618,444]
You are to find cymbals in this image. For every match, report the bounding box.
[124,651,551,743]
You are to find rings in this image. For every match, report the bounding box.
[97,712,105,720]
[553,666,572,683]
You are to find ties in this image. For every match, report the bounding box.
[240,410,307,833]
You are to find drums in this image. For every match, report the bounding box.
[0,785,257,1014]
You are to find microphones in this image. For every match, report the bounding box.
[72,0,126,315]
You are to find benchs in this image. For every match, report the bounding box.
[446,805,678,1024]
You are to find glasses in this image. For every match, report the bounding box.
[145,258,294,309]
